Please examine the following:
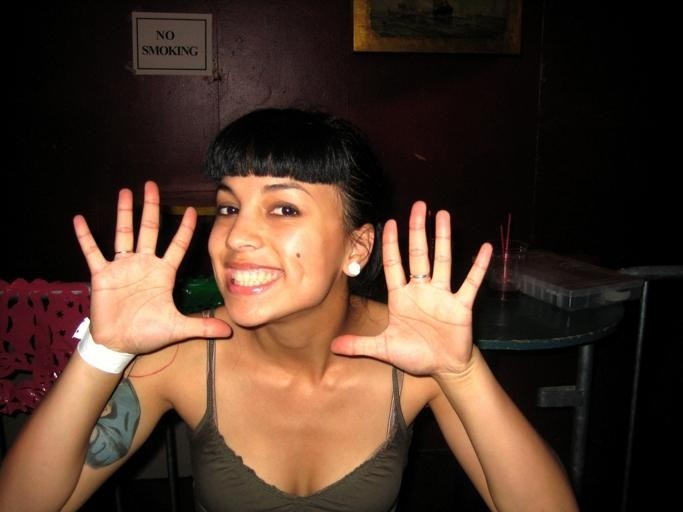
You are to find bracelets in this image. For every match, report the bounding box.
[70,315,136,374]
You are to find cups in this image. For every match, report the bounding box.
[481,247,524,310]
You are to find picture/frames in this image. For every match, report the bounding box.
[353,0,523,54]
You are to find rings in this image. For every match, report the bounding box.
[409,272,430,279]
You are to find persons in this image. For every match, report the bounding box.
[1,109,583,512]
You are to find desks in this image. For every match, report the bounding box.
[475,292,625,498]
[135,192,218,216]
